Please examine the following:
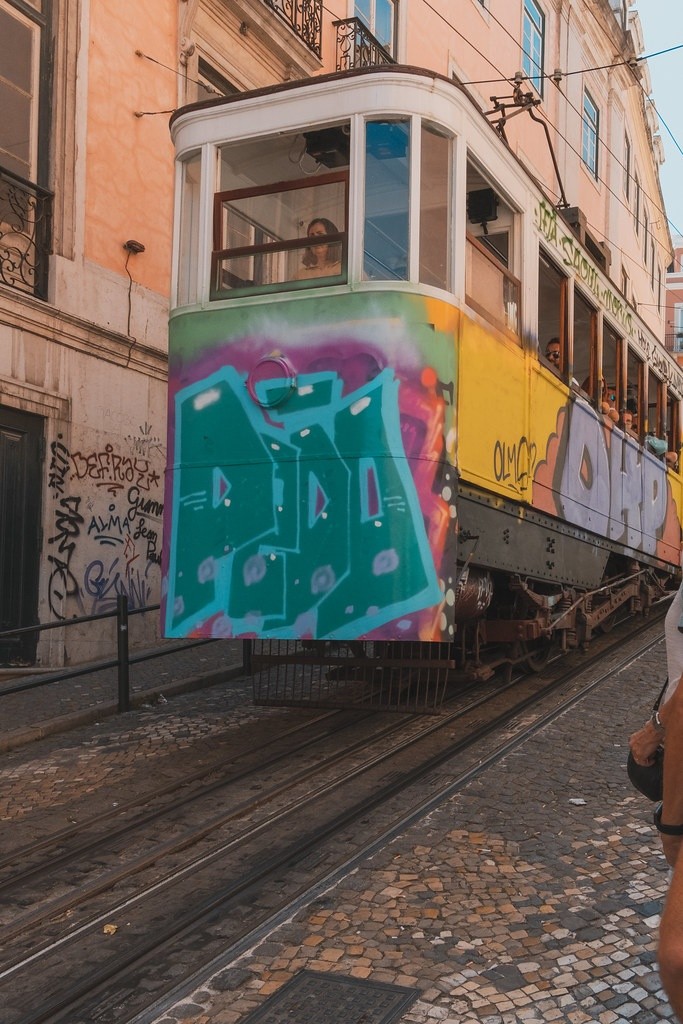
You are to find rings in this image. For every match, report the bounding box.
[634,757,639,761]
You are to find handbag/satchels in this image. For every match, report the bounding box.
[627,674,668,802]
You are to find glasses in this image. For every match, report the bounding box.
[543,350,560,358]
[610,394,616,402]
[602,387,605,392]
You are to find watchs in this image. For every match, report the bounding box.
[651,710,665,736]
[653,800,683,836]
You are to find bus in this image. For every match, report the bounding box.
[168,62,683,716]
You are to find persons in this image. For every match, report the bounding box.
[629,578,683,1024]
[546,337,634,431]
[290,217,368,281]
